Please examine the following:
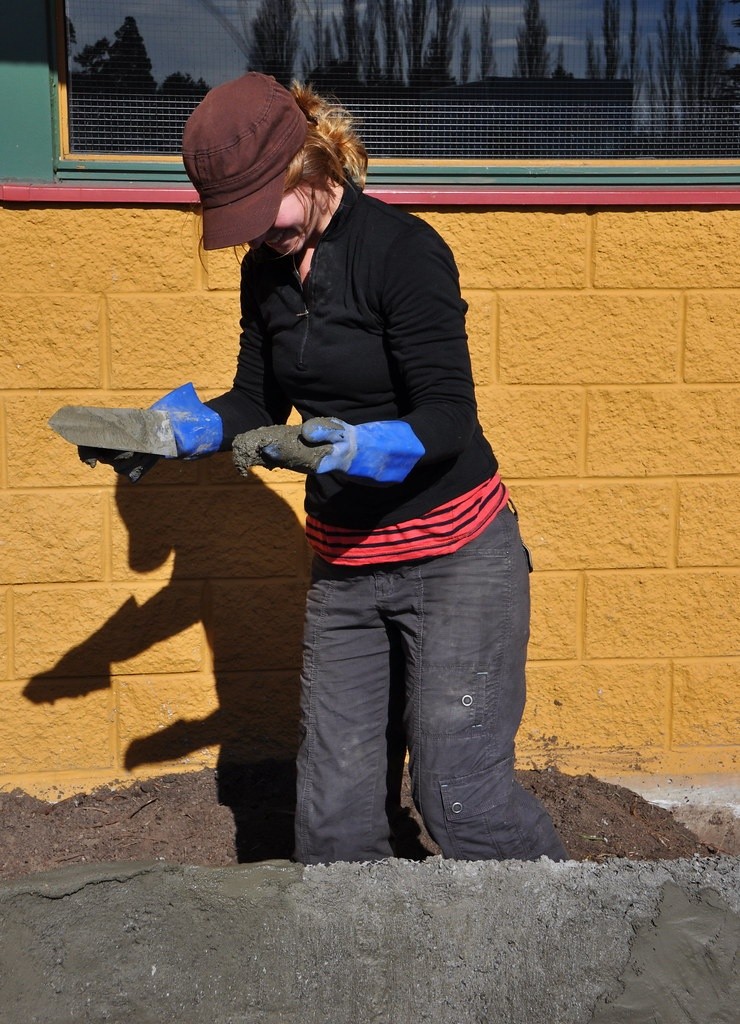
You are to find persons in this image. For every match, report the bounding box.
[78,67,572,864]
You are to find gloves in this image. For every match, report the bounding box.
[78,382,223,483]
[231,416,426,484]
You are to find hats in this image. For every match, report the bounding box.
[182,73,308,250]
